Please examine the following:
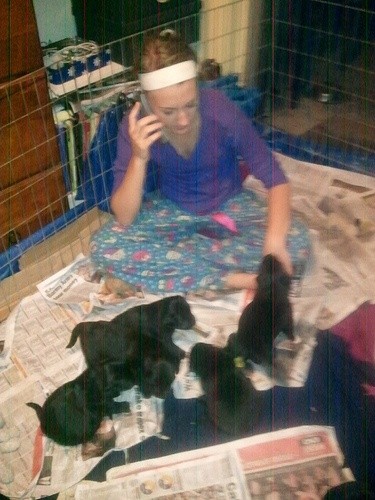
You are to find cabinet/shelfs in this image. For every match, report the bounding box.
[0,0,69,256]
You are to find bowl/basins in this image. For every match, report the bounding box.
[315,91,332,102]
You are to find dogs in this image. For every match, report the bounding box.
[231,253,297,369]
[25,295,262,446]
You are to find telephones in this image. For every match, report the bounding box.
[136,95,170,145]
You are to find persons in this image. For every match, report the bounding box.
[89,37,312,292]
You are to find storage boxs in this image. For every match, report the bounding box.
[41,38,113,86]
